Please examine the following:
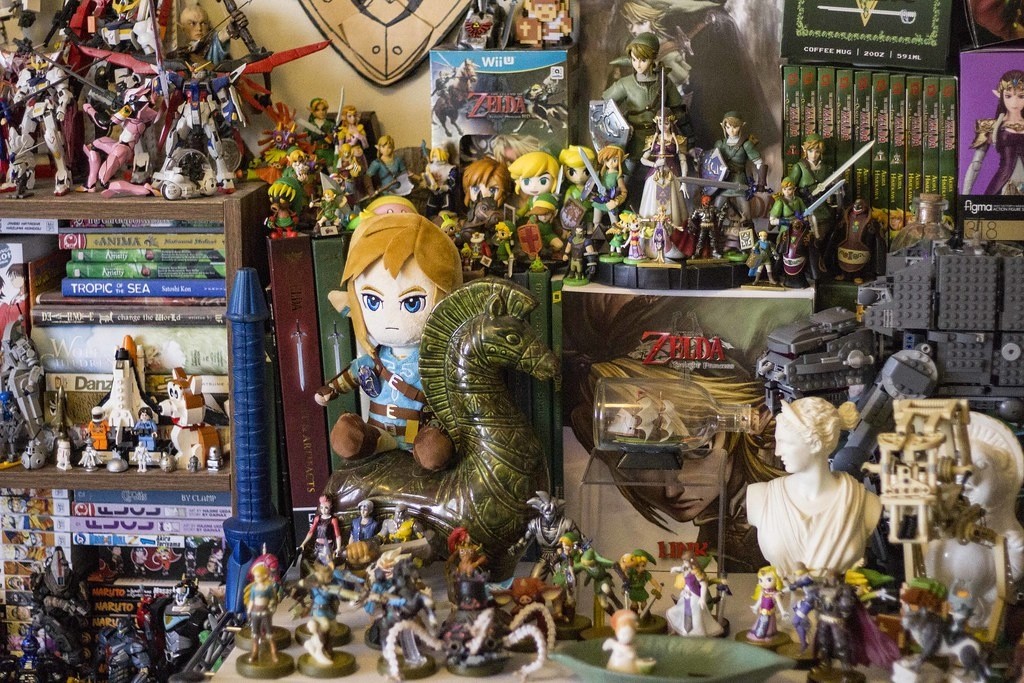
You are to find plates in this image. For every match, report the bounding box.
[546,634,798,683]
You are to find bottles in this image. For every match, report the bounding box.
[592,376,761,452]
[889,193,952,253]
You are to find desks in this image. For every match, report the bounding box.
[208,561,920,683]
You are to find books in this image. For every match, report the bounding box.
[0,490,232,654]
[44,219,225,422]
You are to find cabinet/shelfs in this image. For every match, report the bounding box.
[0,174,271,683]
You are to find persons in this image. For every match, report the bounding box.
[669,551,724,638]
[243,493,438,663]
[750,566,782,639]
[78,407,158,472]
[0,0,251,198]
[508,489,623,622]
[747,397,884,574]
[619,549,662,621]
[446,534,487,606]
[1,544,240,683]
[772,558,896,678]
[238,32,907,286]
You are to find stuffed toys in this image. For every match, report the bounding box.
[314,212,464,468]
